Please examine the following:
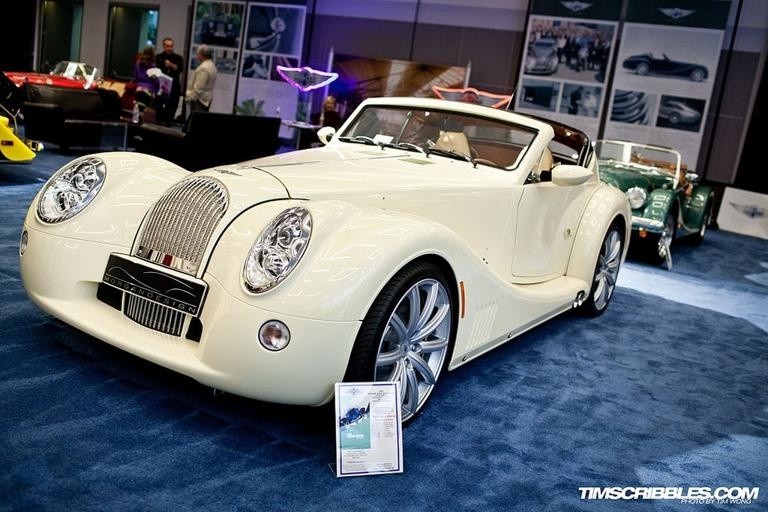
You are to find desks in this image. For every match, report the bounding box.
[279,119,321,149]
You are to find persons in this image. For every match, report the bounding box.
[307,95,342,136]
[527,19,612,83]
[184,44,219,133]
[581,91,598,117]
[152,37,185,125]
[132,46,163,114]
[565,85,584,115]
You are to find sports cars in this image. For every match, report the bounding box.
[525,39,559,75]
[623,51,709,81]
[19,94,632,431]
[6,62,147,92]
[594,138,716,264]
[658,100,700,125]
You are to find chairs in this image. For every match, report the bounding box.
[532,146,553,172]
[435,119,471,159]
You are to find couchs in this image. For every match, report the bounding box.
[139,110,281,172]
[24,83,140,153]
